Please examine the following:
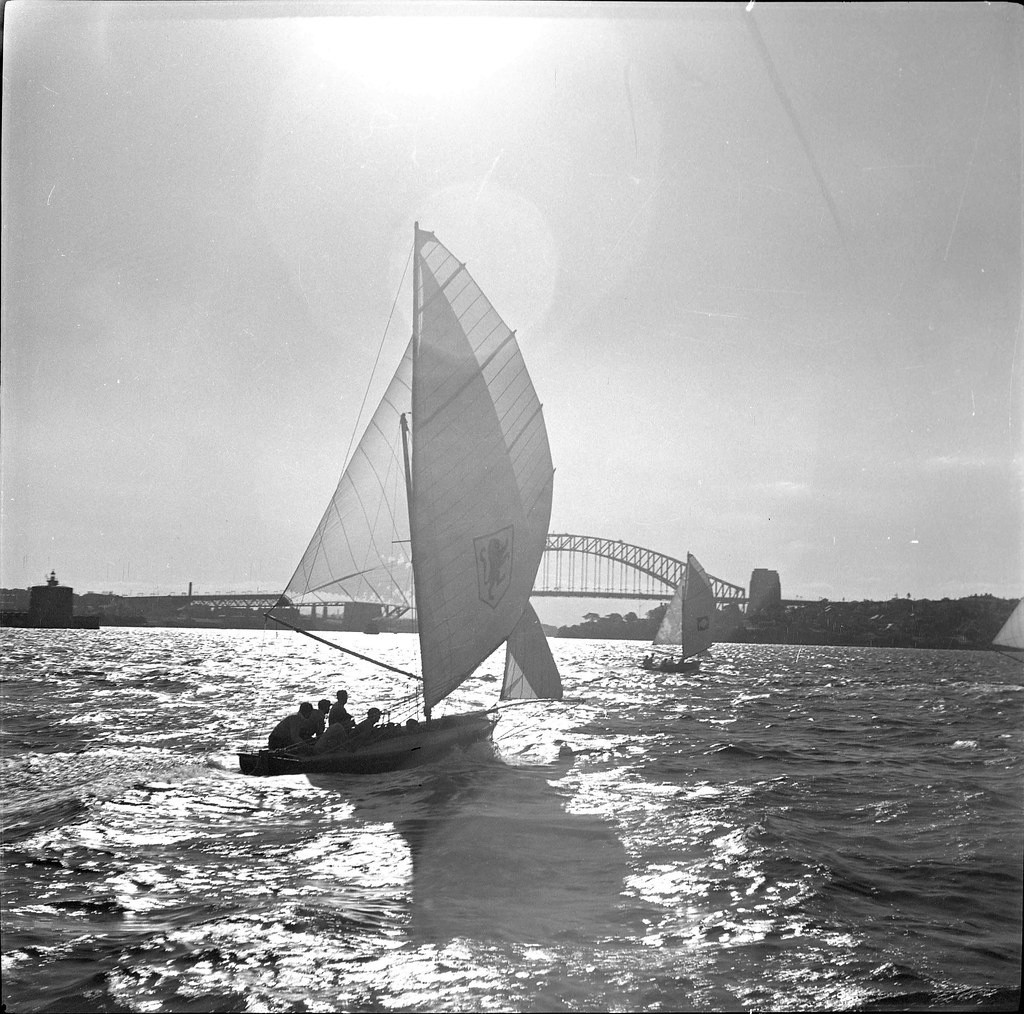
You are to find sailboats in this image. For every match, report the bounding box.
[636,550,716,675]
[233,219,565,777]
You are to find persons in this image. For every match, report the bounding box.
[644,653,655,665]
[305,699,332,738]
[312,714,354,754]
[661,658,667,665]
[329,689,354,728]
[668,657,675,664]
[354,707,381,736]
[269,702,313,747]
